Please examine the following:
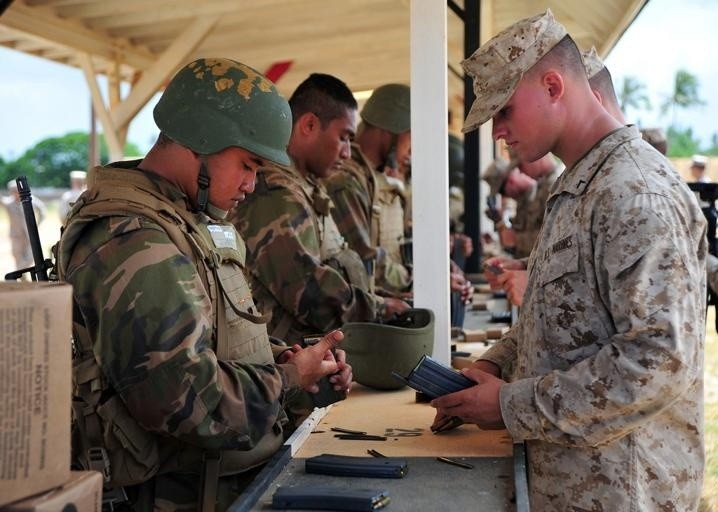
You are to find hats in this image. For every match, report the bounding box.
[484,156,510,196]
[581,45,605,78]
[504,146,519,175]
[456,4,569,135]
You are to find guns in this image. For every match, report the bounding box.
[3,175,53,280]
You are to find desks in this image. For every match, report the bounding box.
[224,278,533,512]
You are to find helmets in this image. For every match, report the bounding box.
[336,303,438,391]
[360,83,415,136]
[151,53,298,167]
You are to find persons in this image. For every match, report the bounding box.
[324,82,474,302]
[229,72,411,443]
[484,48,626,305]
[0,178,47,285]
[58,170,89,227]
[429,9,709,512]
[689,156,707,183]
[49,58,353,512]
[641,127,666,155]
